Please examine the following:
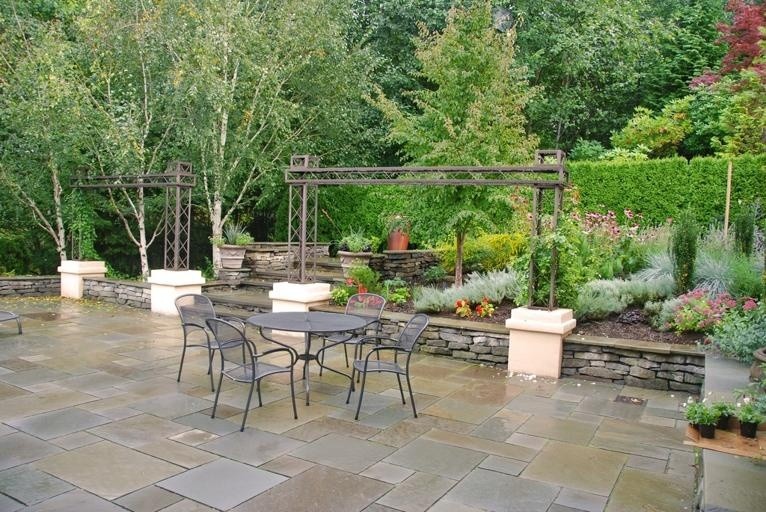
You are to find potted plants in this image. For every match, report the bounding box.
[682,396,764,440]
[206,222,255,269]
[376,205,415,249]
[334,225,380,279]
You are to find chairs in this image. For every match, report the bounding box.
[174,293,429,434]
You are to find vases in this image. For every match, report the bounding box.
[748,347,765,394]
